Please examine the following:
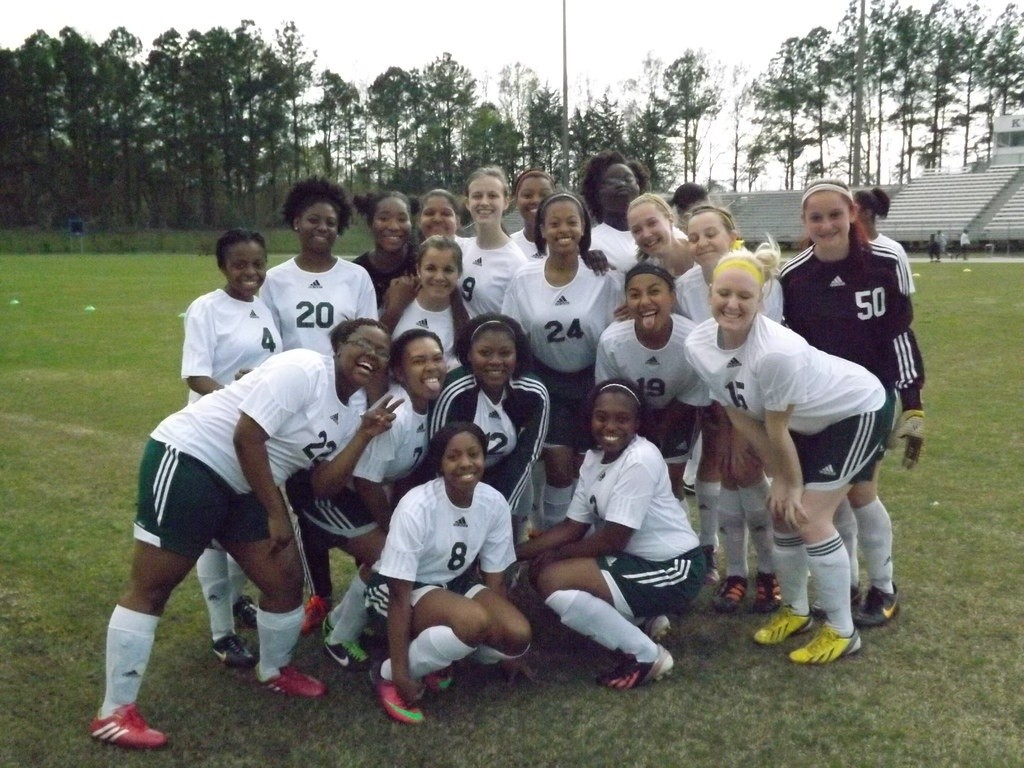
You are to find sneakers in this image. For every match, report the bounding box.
[698,535,720,586]
[713,575,748,612]
[257,665,326,699]
[91,702,167,749]
[753,606,813,646]
[322,639,371,674]
[421,661,454,692]
[232,594,261,628]
[636,614,672,642]
[299,594,333,633]
[210,633,256,668]
[322,613,376,639]
[595,642,677,692]
[754,567,782,613]
[853,581,899,628]
[788,620,861,666]
[367,660,427,726]
[811,579,863,616]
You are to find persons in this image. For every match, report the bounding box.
[581,149,926,663]
[91,174,405,749]
[284,162,706,725]
[934,230,953,259]
[928,242,942,263]
[955,229,971,260]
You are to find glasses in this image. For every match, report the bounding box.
[344,337,391,365]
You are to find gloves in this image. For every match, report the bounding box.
[886,409,926,469]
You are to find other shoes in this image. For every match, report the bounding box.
[508,559,532,602]
